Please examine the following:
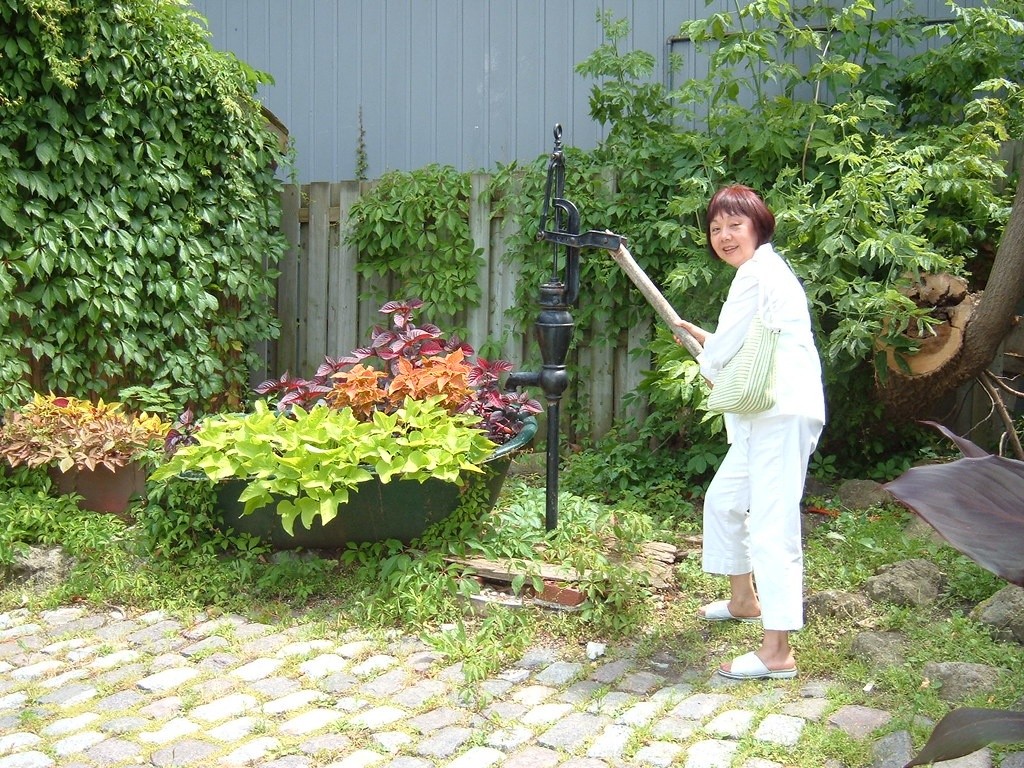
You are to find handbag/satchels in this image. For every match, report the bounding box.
[707,259,782,414]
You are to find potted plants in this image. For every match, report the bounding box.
[0,381,163,522]
[146,296,542,558]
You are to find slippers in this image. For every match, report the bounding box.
[696,600,763,620]
[719,650,798,679]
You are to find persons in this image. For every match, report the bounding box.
[673,185,827,680]
